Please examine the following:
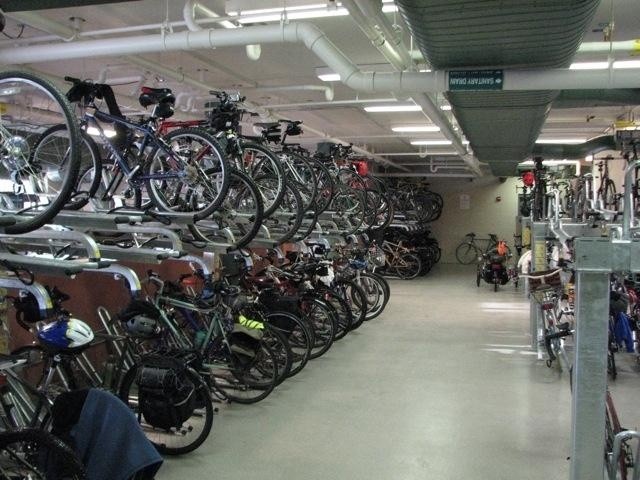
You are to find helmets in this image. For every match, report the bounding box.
[37,319,95,351]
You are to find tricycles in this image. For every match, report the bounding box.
[477,248,518,291]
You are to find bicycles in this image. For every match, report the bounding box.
[546,322,640,480]
[522,123,640,222]
[456,233,511,265]
[518,236,640,380]
